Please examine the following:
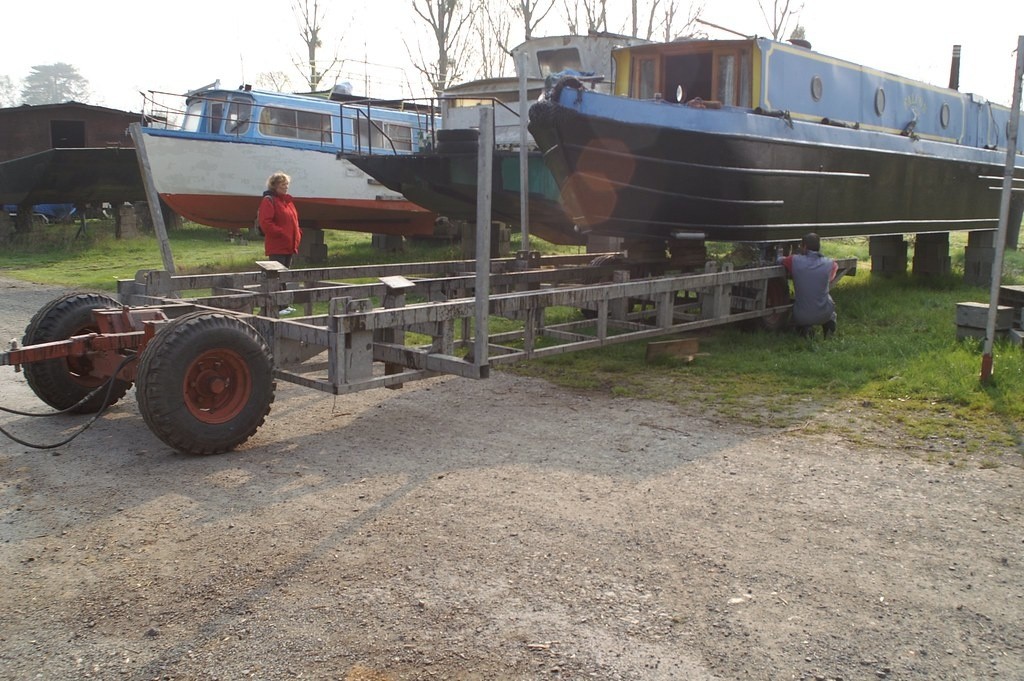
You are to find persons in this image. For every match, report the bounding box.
[776,232,837,338]
[259,173,302,314]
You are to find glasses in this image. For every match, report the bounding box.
[799,243,805,248]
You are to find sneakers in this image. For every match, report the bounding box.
[278,309,291,316]
[284,307,297,312]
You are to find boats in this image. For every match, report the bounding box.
[527,37,1023,238]
[339,32,661,246]
[124,86,461,238]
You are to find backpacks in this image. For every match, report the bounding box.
[256,195,274,237]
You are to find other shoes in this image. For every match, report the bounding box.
[823,328,834,340]
[803,328,816,341]
